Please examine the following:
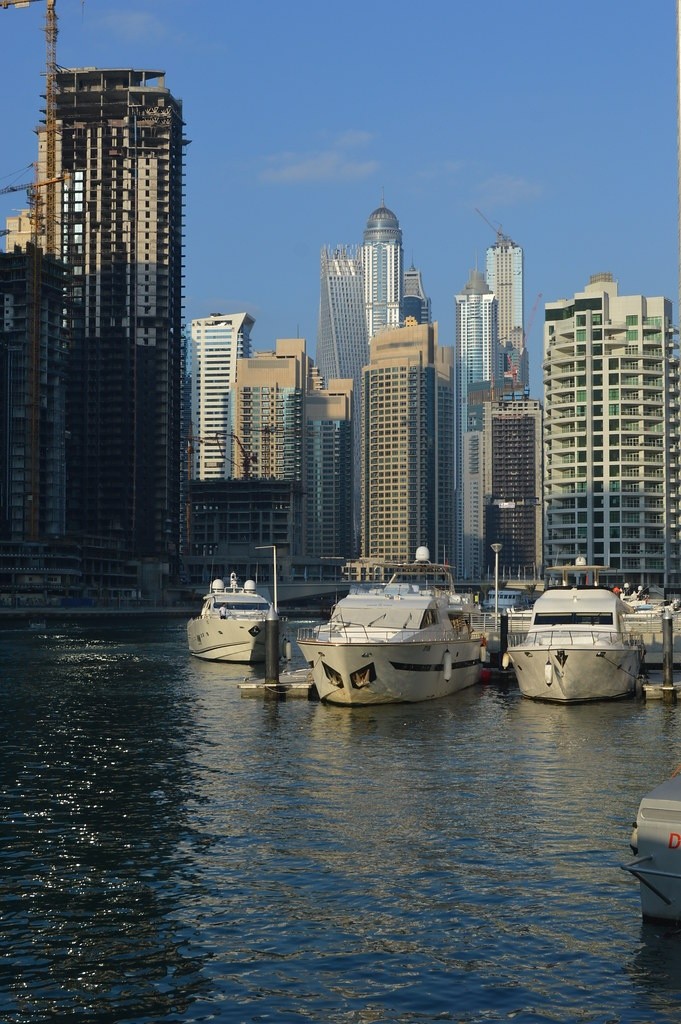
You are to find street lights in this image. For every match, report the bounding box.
[254,546,279,615]
[491,542,503,631]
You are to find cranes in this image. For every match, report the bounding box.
[1,1,61,256]
[474,208,518,249]
[500,291,543,400]
[0,163,72,546]
[189,420,206,479]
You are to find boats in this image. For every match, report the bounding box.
[293,543,489,705]
[186,572,278,665]
[507,558,643,704]
[483,589,523,610]
